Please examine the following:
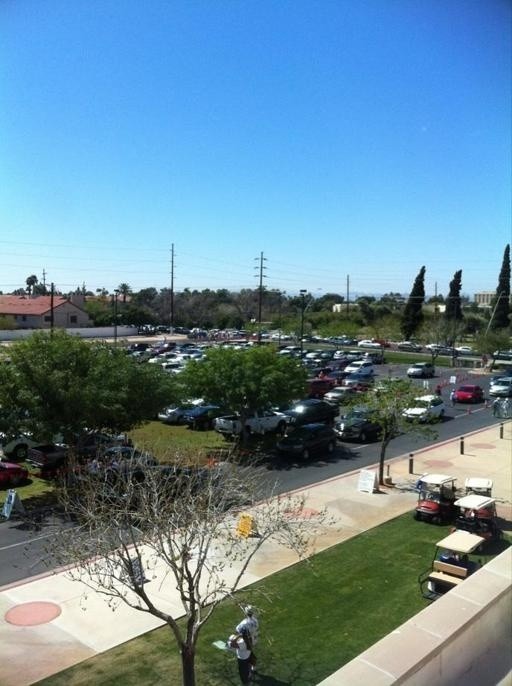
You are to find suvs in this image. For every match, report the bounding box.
[401,394,446,424]
[407,362,435,379]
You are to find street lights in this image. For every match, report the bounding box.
[113,287,120,350]
[299,289,308,368]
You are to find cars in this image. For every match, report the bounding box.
[455,384,483,403]
[271,333,391,350]
[0,425,255,531]
[127,324,271,340]
[122,342,399,458]
[397,341,511,363]
[487,375,512,398]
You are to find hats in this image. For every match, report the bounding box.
[244,605,255,615]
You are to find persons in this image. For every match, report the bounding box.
[434,385,441,395]
[235,605,260,675]
[449,389,456,408]
[231,624,253,686]
[502,397,510,419]
[491,397,501,417]
